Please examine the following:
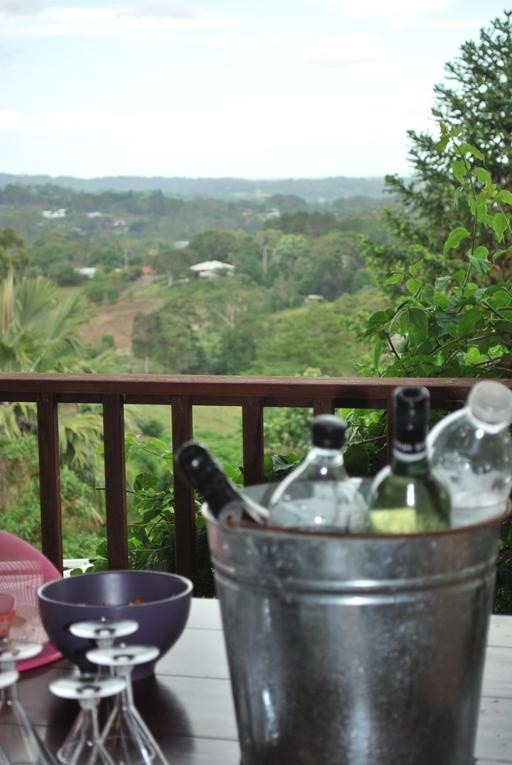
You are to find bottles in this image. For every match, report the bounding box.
[373,382,453,534]
[172,441,271,527]
[427,381,512,532]
[269,414,371,533]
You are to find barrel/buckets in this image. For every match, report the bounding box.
[197,482,512,765]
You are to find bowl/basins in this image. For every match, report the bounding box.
[39,570,193,679]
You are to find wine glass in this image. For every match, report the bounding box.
[1,617,162,765]
